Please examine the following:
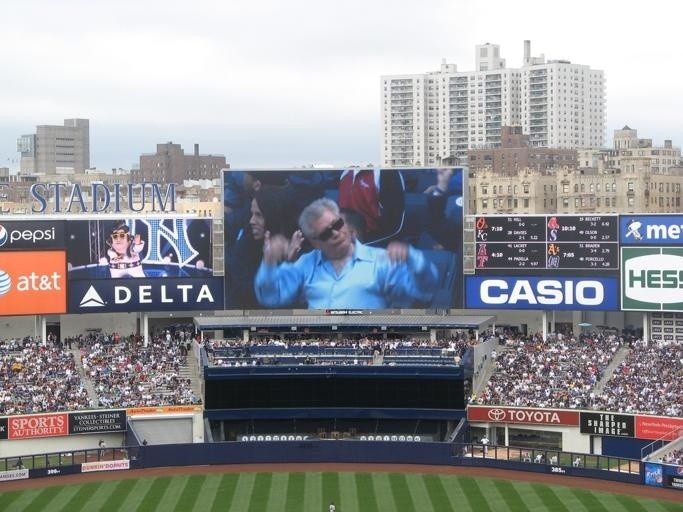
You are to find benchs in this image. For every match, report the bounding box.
[138,346,193,399]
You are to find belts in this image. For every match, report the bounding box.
[109,260,142,269]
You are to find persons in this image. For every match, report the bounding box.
[102,223,147,279]
[550,454,557,464]
[204,331,475,366]
[15,457,22,468]
[481,435,489,454]
[657,447,683,466]
[223,169,463,307]
[99,439,106,456]
[142,438,147,445]
[466,322,682,419]
[572,455,580,468]
[540,453,545,463]
[0,323,199,414]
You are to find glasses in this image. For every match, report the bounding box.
[110,234,128,239]
[308,216,344,242]
